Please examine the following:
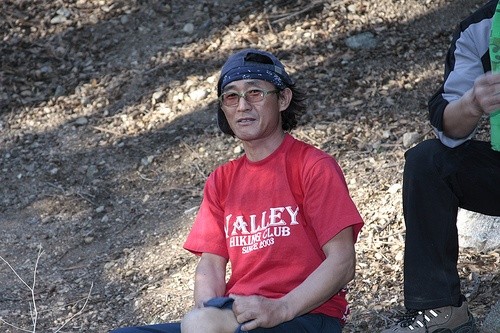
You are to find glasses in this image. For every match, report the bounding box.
[219,88,280,108]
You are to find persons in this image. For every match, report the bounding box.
[106,46,364,333]
[379,0,500,332]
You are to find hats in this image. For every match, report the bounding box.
[216,49,291,135]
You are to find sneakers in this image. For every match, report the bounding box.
[378,297,479,332]
